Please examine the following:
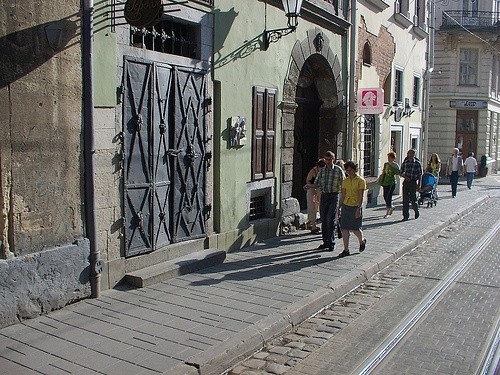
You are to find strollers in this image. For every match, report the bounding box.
[417,168,439,208]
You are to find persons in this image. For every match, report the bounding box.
[303,150,348,251]
[338,160,366,257]
[381,152,400,219]
[445,147,464,198]
[464,151,478,189]
[428,153,441,199]
[400,149,423,221]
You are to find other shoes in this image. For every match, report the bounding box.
[415,211,419,219]
[319,244,328,249]
[402,217,408,221]
[338,250,349,257]
[311,227,322,234]
[384,214,390,218]
[453,196,455,198]
[390,206,395,215]
[359,238,366,251]
[329,245,334,250]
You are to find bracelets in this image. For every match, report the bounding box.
[357,206,361,208]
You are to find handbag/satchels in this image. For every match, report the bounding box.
[376,173,385,186]
